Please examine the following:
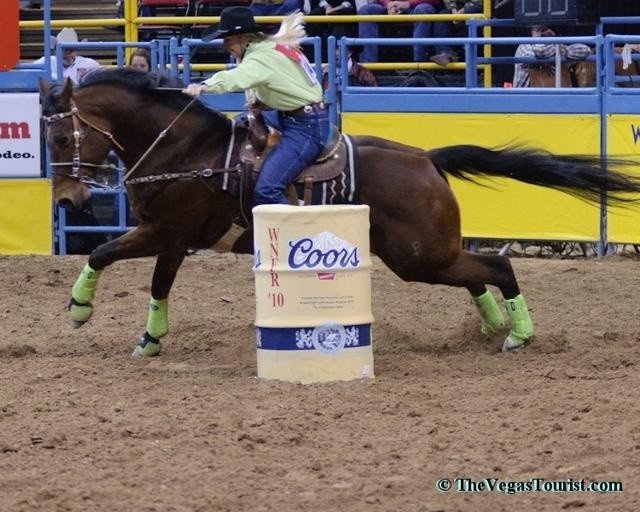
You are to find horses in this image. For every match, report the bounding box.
[38,66,640,359]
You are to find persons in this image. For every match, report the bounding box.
[180,5,332,206]
[34,25,101,84]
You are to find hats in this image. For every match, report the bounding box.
[200,6,263,42]
[50,27,88,49]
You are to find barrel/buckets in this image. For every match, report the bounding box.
[250,200,377,387]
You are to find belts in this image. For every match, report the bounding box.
[280,101,325,118]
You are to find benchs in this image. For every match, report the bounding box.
[18,2,597,87]
